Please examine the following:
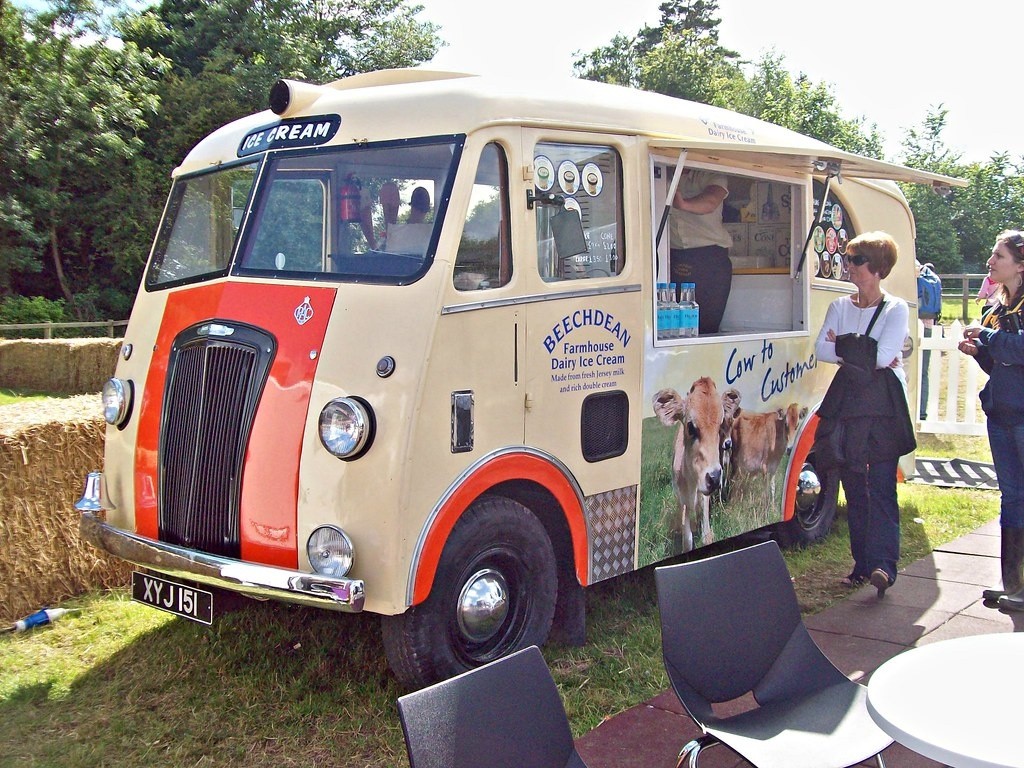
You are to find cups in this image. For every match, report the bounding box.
[537,167,549,189]
[587,173,598,195]
[563,170,575,192]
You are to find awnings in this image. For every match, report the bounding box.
[649,139,968,190]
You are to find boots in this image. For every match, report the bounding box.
[983,526,1024,611]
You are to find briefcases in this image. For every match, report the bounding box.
[813,417,848,473]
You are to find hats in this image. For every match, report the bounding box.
[379,182,400,207]
[411,187,430,212]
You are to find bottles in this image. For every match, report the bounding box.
[657,282,679,340]
[679,283,692,338]
[686,283,699,337]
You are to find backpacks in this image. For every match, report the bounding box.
[917,265,942,319]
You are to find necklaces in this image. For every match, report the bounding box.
[856,293,883,334]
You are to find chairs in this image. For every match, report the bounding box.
[656,539,896,768]
[396,645,589,768]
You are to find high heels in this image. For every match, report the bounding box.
[870,569,889,598]
[841,573,865,586]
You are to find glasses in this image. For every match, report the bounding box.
[847,255,868,266]
[1010,233,1024,256]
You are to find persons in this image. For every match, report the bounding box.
[958,230,1024,612]
[815,231,908,600]
[667,166,733,335]
[916,258,939,419]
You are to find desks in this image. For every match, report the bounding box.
[866,632,1024,768]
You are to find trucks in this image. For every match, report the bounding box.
[74,68,971,689]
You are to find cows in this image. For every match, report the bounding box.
[651,375,809,552]
